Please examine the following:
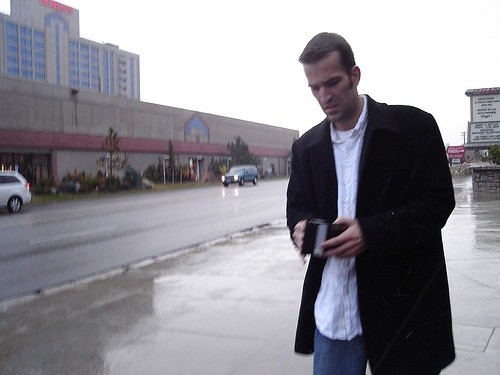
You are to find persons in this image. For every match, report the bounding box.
[285,32,456,375]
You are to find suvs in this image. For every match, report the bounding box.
[221,164,260,187]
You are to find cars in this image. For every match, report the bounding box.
[0,172,31,214]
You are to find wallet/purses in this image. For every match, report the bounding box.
[301,217,348,256]
